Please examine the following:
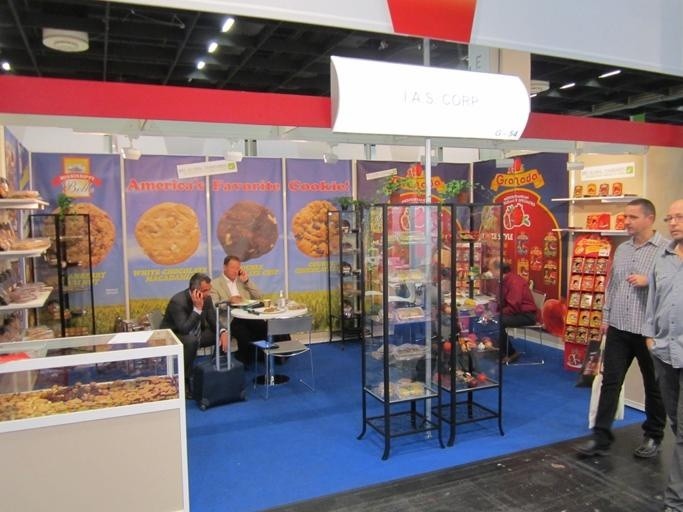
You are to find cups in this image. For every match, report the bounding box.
[287,303,297,310]
[263,299,271,307]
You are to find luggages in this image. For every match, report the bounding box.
[192,301,248,412]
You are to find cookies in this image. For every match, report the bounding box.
[135,203,200,266]
[216,200,279,262]
[43,204,114,270]
[290,200,340,258]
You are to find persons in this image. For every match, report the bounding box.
[159,273,228,399]
[475,257,537,364]
[642,199,683,512]
[577,199,672,458]
[210,255,267,370]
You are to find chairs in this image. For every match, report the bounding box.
[506,290,546,366]
[253,318,315,400]
[388,281,416,343]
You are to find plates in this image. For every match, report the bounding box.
[230,299,260,307]
[253,307,286,314]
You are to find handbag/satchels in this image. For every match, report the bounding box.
[230,302,264,316]
[573,332,604,388]
[588,373,624,429]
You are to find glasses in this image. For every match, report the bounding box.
[663,215,683,223]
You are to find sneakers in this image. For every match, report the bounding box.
[497,351,519,364]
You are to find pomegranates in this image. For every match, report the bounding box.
[504,205,524,229]
[543,296,567,336]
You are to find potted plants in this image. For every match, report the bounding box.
[383,175,416,204]
[447,179,472,205]
[352,199,368,210]
[57,193,77,221]
[338,197,351,210]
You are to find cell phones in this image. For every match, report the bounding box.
[238,270,243,276]
[190,287,201,298]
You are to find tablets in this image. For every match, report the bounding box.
[251,339,280,350]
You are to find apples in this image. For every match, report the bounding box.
[478,374,488,382]
[445,307,451,315]
[442,342,452,351]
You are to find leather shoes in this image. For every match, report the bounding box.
[634,436,663,458]
[570,433,613,456]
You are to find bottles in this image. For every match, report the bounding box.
[277,289,287,310]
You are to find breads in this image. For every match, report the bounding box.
[7,190,39,198]
[11,239,49,250]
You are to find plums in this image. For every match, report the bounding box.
[470,379,477,387]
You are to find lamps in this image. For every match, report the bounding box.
[224,139,242,162]
[121,136,142,160]
[324,142,338,164]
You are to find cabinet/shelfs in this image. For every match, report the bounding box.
[419,202,505,446]
[551,195,637,236]
[0,328,189,512]
[356,203,445,460]
[29,214,96,387]
[0,198,54,391]
[326,210,373,351]
